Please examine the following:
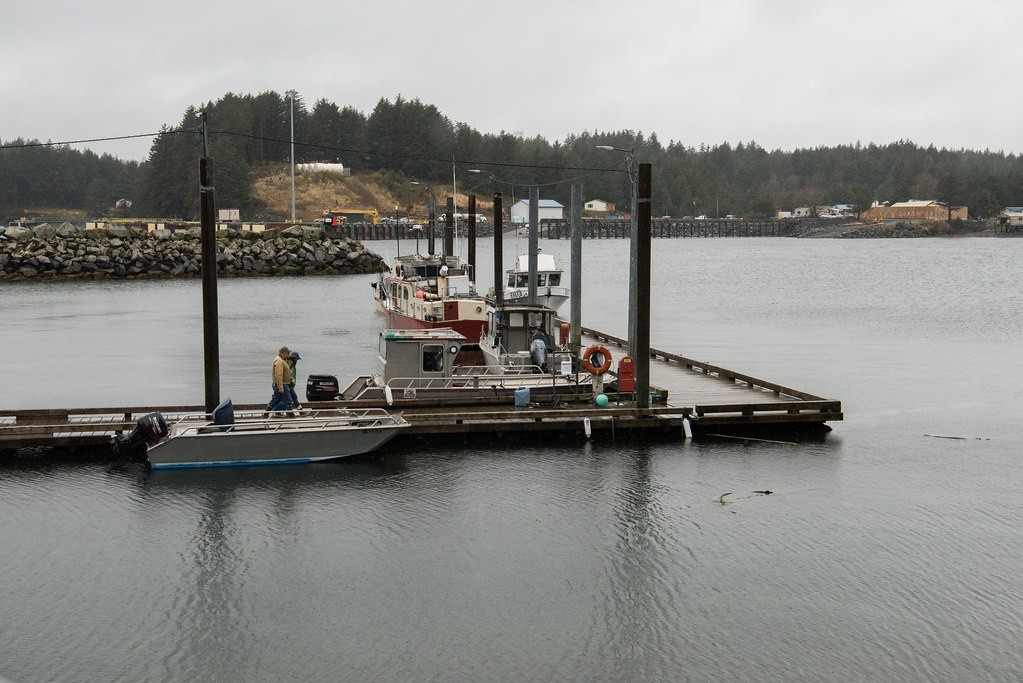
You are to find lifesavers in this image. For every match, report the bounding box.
[583,345,612,375]
[396,265,400,276]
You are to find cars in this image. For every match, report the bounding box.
[438,213,486,223]
[662,215,737,219]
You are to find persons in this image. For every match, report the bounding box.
[270,352,310,419]
[262,347,300,426]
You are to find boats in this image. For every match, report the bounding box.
[487,185,569,314]
[111,398,411,469]
[305,325,618,407]
[371,154,490,344]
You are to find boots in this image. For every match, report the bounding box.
[299,408,310,416]
[263,405,272,418]
[286,410,295,418]
[274,412,285,417]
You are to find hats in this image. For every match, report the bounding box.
[279,347,292,354]
[291,351,301,359]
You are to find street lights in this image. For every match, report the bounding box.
[289,89,297,223]
[595,145,651,419]
